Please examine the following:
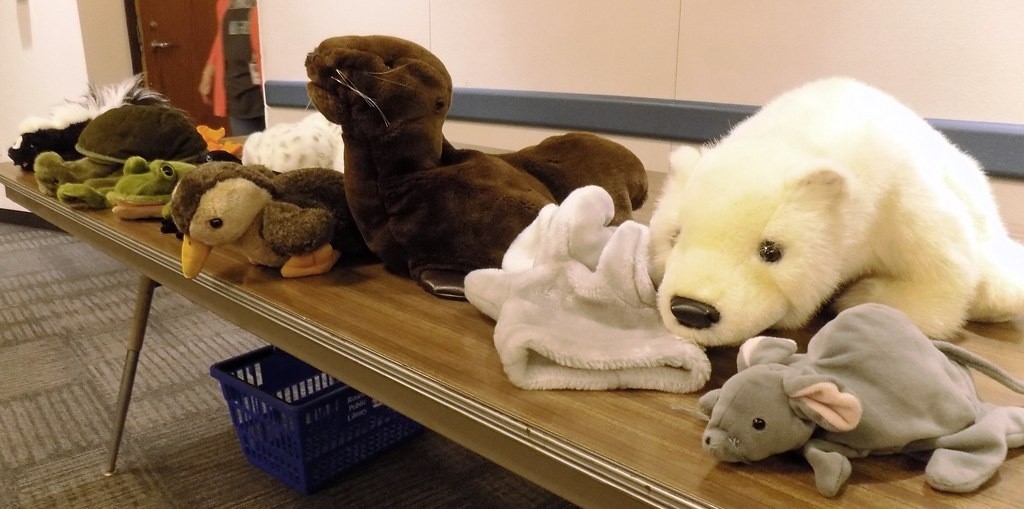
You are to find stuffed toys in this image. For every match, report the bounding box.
[646,77,1024,346]
[34,105,209,209]
[106,156,197,219]
[170,161,348,278]
[305,37,649,269]
[242,113,345,173]
[196,126,243,158]
[698,303,1024,498]
[8,73,166,171]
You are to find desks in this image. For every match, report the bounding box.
[0,161,1024,509]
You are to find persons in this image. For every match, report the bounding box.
[198,0,262,136]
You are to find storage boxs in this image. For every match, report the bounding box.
[209,343,429,493]
